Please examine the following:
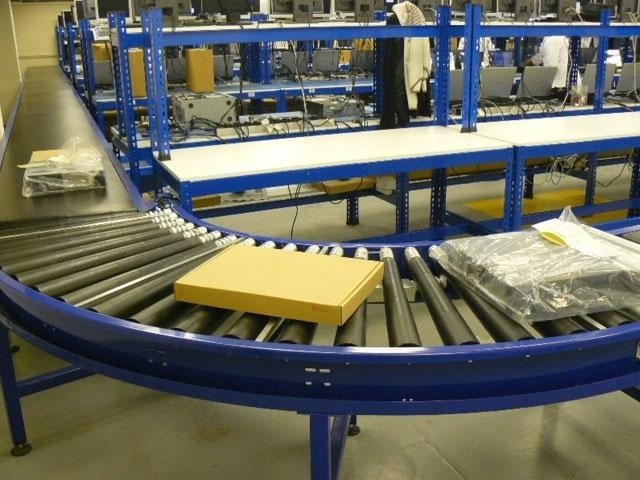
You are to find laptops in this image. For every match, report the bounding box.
[450,70,464,101]
[616,62,640,92]
[479,66,518,99]
[450,47,640,70]
[93,49,374,85]
[516,65,559,99]
[582,63,617,94]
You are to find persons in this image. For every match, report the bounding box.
[458,37,495,65]
[524,36,571,88]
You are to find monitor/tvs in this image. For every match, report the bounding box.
[58,0,640,27]
